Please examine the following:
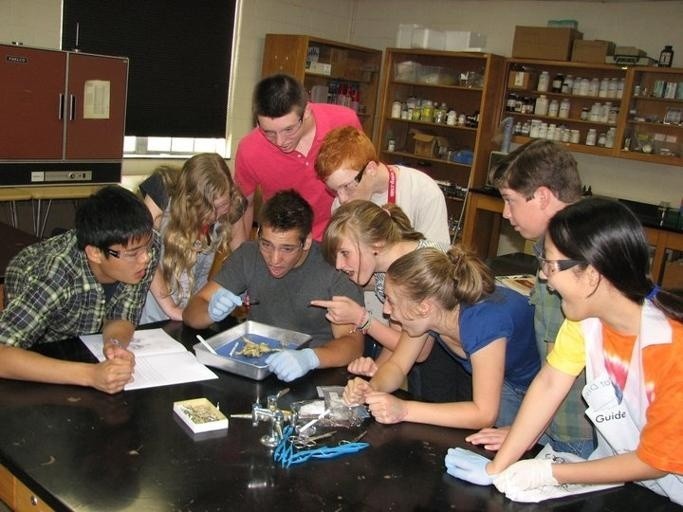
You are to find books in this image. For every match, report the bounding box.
[494,272,538,296]
[76,328,220,391]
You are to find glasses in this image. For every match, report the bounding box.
[532,235,582,278]
[107,234,155,261]
[255,229,303,254]
[215,196,234,211]
[256,101,307,137]
[326,161,369,196]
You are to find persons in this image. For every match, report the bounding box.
[343,246,553,449]
[444,194,683,506]
[314,125,451,381]
[139,152,247,327]
[229,72,362,257]
[1,186,162,395]
[183,185,365,383]
[311,198,473,402]
[463,139,598,460]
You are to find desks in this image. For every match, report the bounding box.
[463,189,683,288]
[0,315,683,512]
[0,186,104,238]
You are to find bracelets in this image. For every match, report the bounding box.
[351,307,365,332]
[103,337,126,347]
[357,311,373,335]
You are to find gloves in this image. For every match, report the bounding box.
[492,459,557,495]
[265,347,320,383]
[208,287,243,322]
[444,447,495,484]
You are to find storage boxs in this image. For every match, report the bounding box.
[414,134,448,158]
[318,45,372,85]
[571,40,615,64]
[512,25,583,61]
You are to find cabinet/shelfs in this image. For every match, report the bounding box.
[0,43,129,184]
[264,33,382,142]
[493,58,683,166]
[372,46,506,262]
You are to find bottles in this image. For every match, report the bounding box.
[533,71,624,99]
[506,92,619,125]
[387,94,466,128]
[658,46,674,68]
[387,139,395,152]
[510,118,616,148]
